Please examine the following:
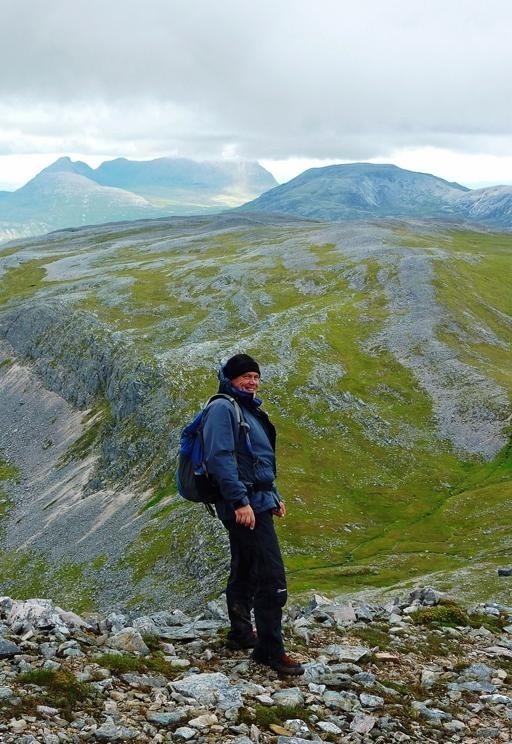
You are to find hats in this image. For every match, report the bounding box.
[222,354,261,380]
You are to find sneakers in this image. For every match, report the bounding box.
[249,643,305,678]
[227,631,259,652]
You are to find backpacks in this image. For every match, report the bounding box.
[175,393,258,506]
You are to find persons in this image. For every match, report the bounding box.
[203,353,306,676]
